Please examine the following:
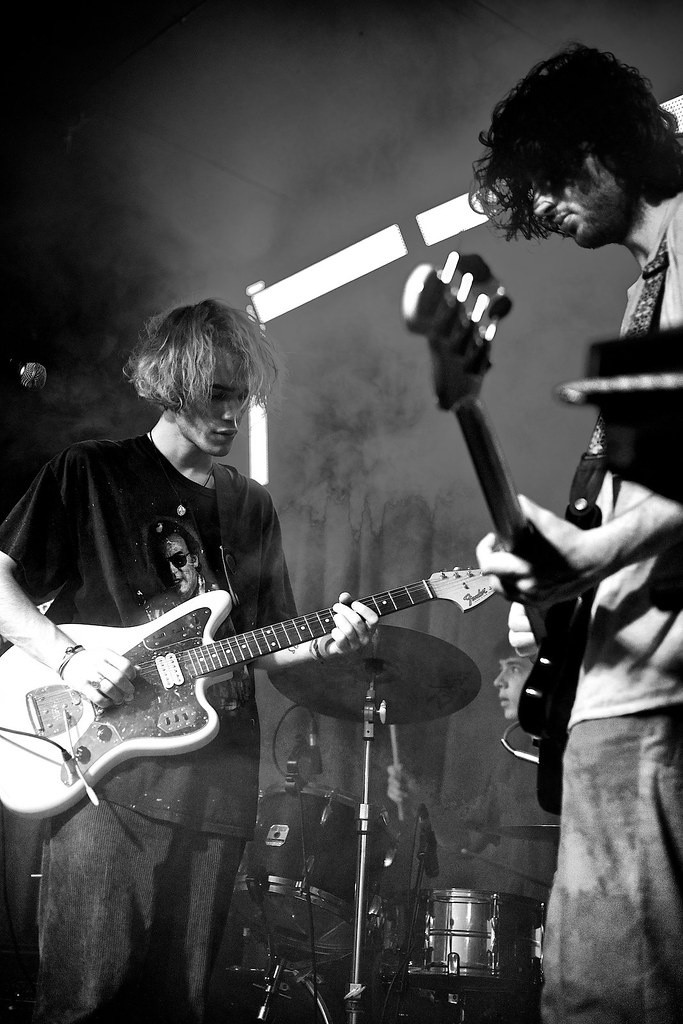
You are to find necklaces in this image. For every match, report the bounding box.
[150,431,214,516]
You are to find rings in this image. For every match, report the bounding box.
[91,677,106,689]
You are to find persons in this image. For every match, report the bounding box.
[387,636,559,909]
[472,42,683,1024]
[133,521,235,642]
[1,299,386,1024]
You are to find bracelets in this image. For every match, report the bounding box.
[56,644,85,676]
[308,638,326,664]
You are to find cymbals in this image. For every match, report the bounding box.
[267,621,480,726]
[497,823,563,842]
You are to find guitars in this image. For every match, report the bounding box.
[2,567,502,816]
[385,250,609,814]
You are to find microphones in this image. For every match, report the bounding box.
[309,718,322,775]
[418,803,439,878]
[0,355,47,391]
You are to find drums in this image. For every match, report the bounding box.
[410,888,546,985]
[223,792,414,967]
